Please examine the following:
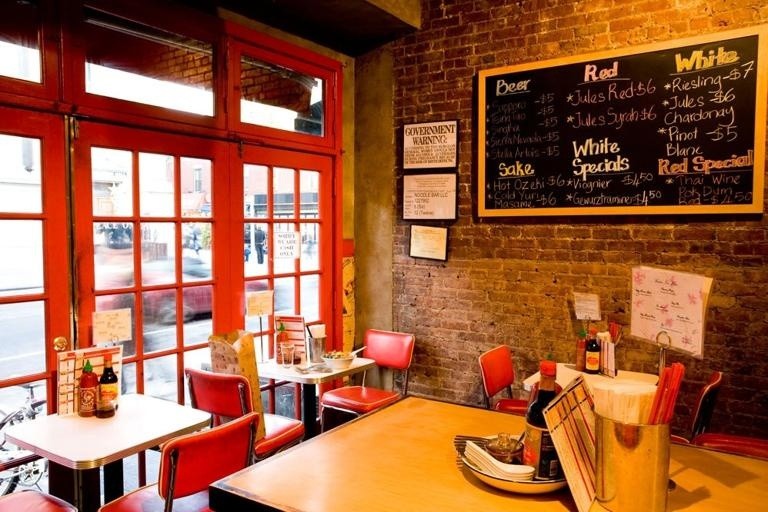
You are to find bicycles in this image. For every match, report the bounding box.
[0,384,48,496]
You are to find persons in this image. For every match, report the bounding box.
[254,226,266,264]
[244,245,252,261]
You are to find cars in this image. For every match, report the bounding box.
[94,258,281,326]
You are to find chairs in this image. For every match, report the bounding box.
[478,345,529,416]
[185,368,305,465]
[97,410,261,511]
[319,328,416,433]
[670,370,724,444]
[1,489,79,512]
[690,432,768,458]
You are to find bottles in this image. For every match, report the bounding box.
[574,328,590,371]
[482,432,526,468]
[519,359,563,482]
[585,325,601,375]
[79,359,98,418]
[274,323,289,364]
[98,353,120,414]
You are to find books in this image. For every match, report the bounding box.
[275,316,307,363]
[57,345,123,416]
[595,337,616,378]
[540,373,598,511]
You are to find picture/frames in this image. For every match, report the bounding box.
[401,119,459,170]
[408,224,449,261]
[401,171,458,221]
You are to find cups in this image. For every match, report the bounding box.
[308,337,327,363]
[293,350,301,364]
[591,410,671,512]
[281,345,294,368]
[95,384,118,419]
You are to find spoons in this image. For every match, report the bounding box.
[295,363,332,374]
[463,440,536,484]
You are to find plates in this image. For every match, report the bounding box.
[458,435,567,495]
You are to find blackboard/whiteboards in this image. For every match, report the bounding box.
[478,24,768,218]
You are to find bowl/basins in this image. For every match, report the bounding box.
[320,353,356,369]
